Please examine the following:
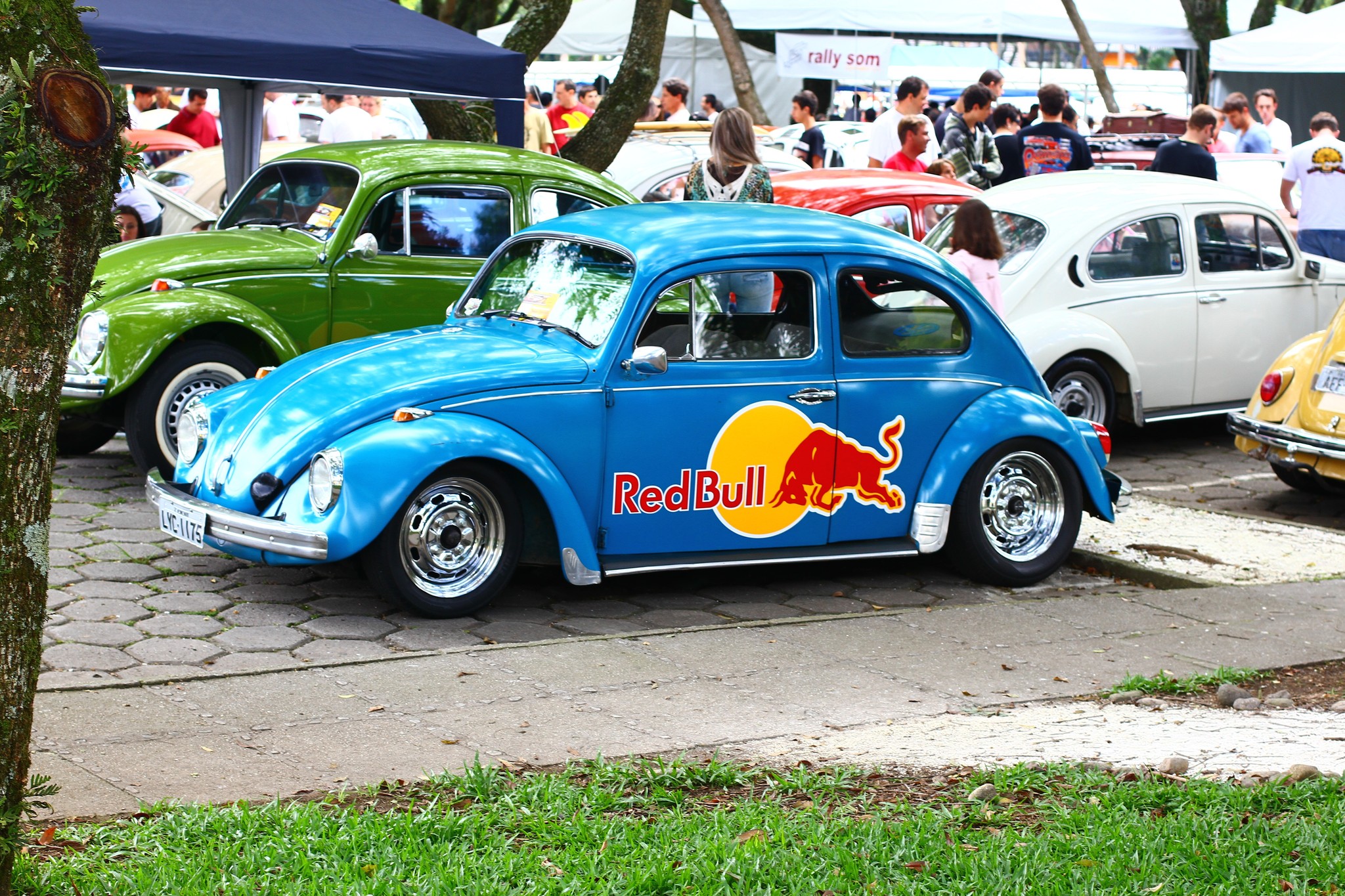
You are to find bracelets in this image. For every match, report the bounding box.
[1291,211,1299,218]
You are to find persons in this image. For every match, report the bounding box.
[1151,104,1219,181]
[192,220,216,231]
[944,198,1003,324]
[1202,107,1229,152]
[318,92,398,145]
[1280,111,1345,262]
[866,69,1095,236]
[1254,88,1292,156]
[791,90,825,170]
[111,205,148,242]
[1223,92,1273,154]
[521,77,725,157]
[261,90,301,141]
[843,93,862,121]
[123,81,223,155]
[108,167,162,238]
[682,108,775,312]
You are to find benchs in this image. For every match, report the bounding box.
[764,322,890,357]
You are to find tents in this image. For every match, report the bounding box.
[1209,0,1345,146]
[66,0,524,198]
[475,0,803,125]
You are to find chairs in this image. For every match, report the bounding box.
[716,339,793,359]
[1132,241,1177,276]
[639,325,739,361]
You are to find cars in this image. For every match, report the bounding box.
[56,122,1345,621]
